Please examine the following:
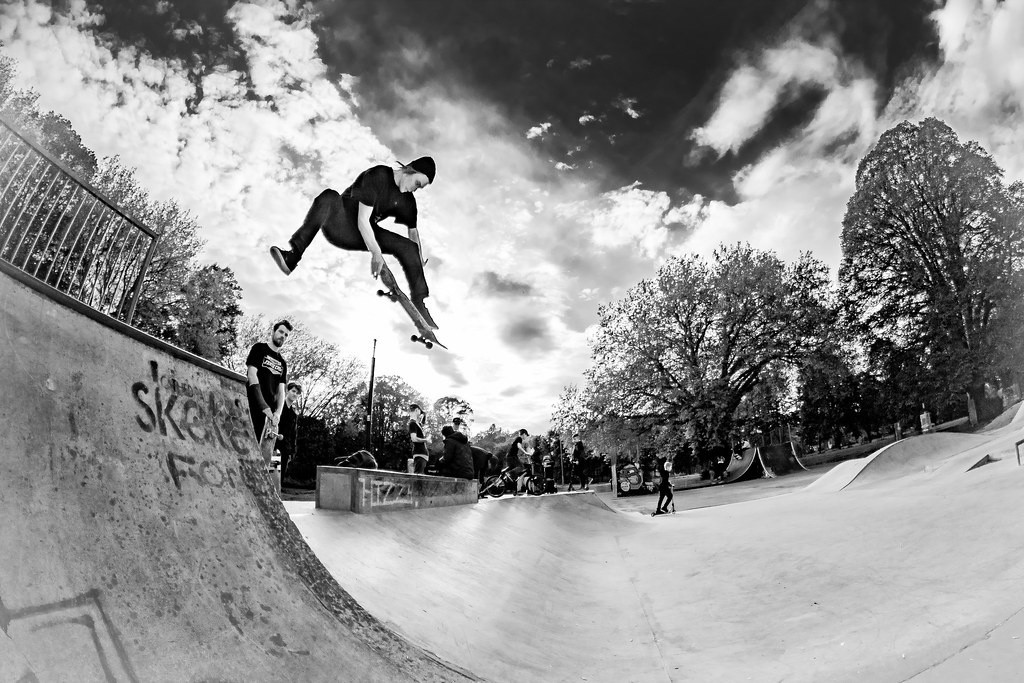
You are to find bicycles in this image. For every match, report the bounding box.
[485,451,548,498]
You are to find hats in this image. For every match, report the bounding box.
[412,157,436,184]
[520,429,529,436]
[453,418,461,422]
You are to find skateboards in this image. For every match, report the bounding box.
[376,264,449,352]
[259,416,284,473]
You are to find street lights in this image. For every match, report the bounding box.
[366,338,377,451]
[555,431,564,486]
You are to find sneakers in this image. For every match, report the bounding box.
[416,303,439,330]
[270,246,298,275]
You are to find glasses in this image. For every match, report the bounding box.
[454,423,460,425]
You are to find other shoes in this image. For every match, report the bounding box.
[656,511,664,514]
[662,507,670,513]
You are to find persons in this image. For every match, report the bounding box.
[270,155,441,330]
[530,440,556,481]
[407,403,433,474]
[571,434,593,490]
[443,417,461,441]
[505,429,530,496]
[709,454,729,484]
[407,432,416,474]
[435,425,474,480]
[272,381,303,491]
[246,320,294,463]
[656,461,675,514]
